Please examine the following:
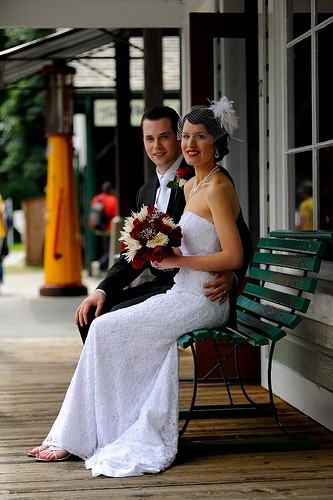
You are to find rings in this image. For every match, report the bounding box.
[223,290,227,295]
[155,266,157,269]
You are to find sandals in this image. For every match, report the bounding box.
[27,443,74,461]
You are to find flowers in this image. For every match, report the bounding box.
[117,203,183,272]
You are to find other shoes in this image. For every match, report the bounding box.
[91,261,100,278]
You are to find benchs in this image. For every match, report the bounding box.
[176,229,333,442]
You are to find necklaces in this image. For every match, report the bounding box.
[189,166,220,196]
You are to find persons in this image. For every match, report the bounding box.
[89,183,118,277]
[75,105,255,345]
[26,96,243,478]
[0,193,7,284]
[298,183,313,230]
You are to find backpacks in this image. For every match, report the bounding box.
[89,196,111,232]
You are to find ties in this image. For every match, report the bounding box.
[155,177,171,213]
